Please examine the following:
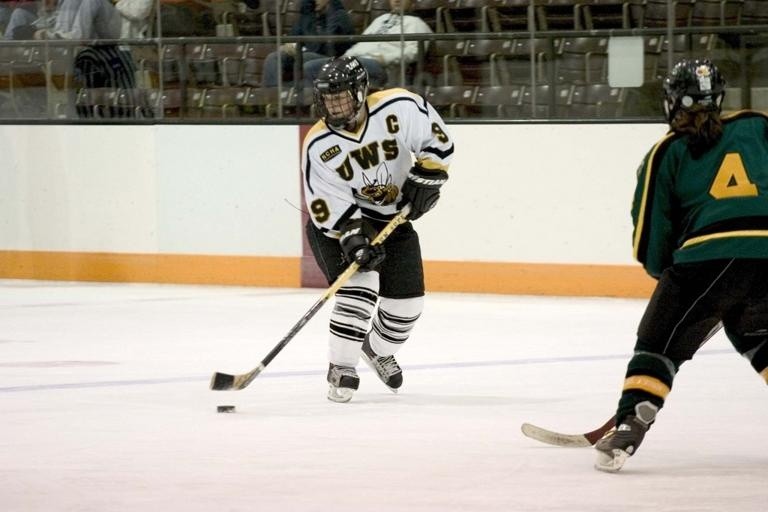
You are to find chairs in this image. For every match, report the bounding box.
[2,0,766,122]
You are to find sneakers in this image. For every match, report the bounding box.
[360,332,404,388]
[326,362,359,391]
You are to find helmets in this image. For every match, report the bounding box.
[663,56,726,109]
[315,55,368,111]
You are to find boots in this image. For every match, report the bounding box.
[594,398,662,456]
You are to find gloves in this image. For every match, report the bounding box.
[395,163,450,221]
[336,218,388,273]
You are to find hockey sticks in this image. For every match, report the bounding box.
[521,315,723,449]
[210,203,411,391]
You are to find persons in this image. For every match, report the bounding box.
[592,59,768,454]
[300,57,455,390]
